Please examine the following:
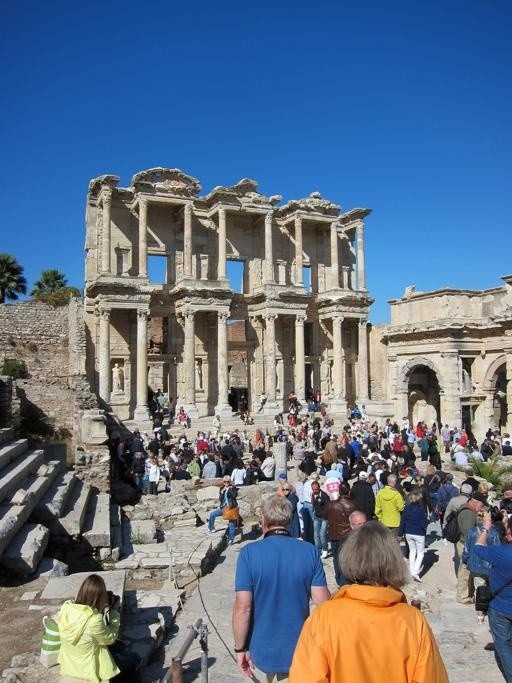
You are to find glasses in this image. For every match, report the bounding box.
[283,488,289,491]
[224,481,229,483]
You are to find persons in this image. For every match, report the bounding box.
[56,574,138,682]
[117,388,512,683]
[112,363,122,393]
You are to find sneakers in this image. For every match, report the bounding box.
[228,539,233,545]
[456,596,474,605]
[322,550,328,558]
[207,528,216,533]
[477,616,484,624]
[411,573,423,582]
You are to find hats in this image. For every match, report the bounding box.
[471,491,489,508]
[359,470,368,479]
[279,474,287,479]
[308,472,319,479]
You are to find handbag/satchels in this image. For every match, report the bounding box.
[223,506,238,520]
[39,614,60,669]
[475,585,492,612]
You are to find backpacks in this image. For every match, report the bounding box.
[389,456,401,473]
[437,485,455,512]
[441,506,473,544]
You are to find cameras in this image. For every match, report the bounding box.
[488,506,503,522]
[105,591,111,604]
